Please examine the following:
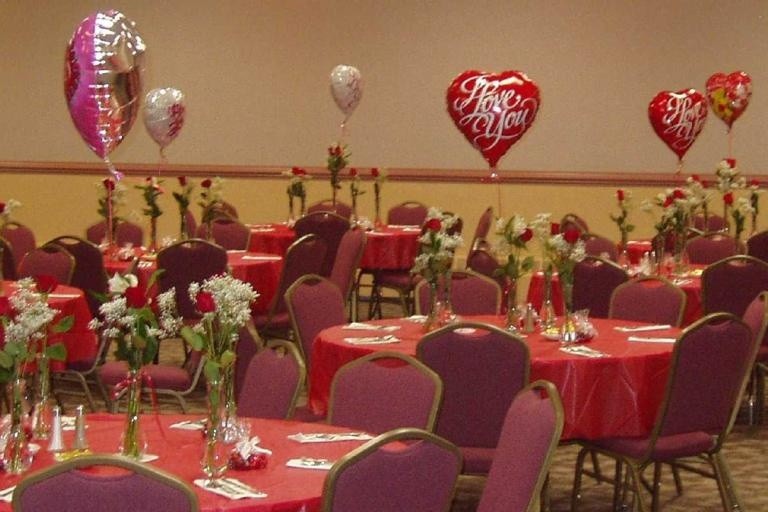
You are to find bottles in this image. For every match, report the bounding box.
[651,251,658,274]
[47,404,67,453]
[523,302,535,332]
[642,251,650,276]
[72,404,89,450]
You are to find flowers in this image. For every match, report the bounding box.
[665,187,709,274]
[348,168,366,223]
[409,205,453,330]
[196,176,238,244]
[180,271,261,470]
[437,212,466,311]
[743,177,767,235]
[0,198,23,223]
[494,211,535,325]
[88,268,184,457]
[286,166,300,215]
[609,190,636,257]
[326,141,353,208]
[533,212,561,304]
[32,272,75,436]
[97,178,128,245]
[687,173,728,239]
[296,168,314,215]
[723,192,756,252]
[544,228,588,336]
[0,275,62,474]
[371,168,384,217]
[172,174,194,241]
[639,193,678,277]
[134,176,165,251]
[701,158,748,238]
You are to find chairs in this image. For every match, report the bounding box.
[616,239,652,266]
[12,454,200,512]
[466,250,513,289]
[201,210,232,225]
[101,349,206,415]
[252,232,329,348]
[0,221,36,268]
[465,205,493,270]
[561,213,589,233]
[203,199,238,220]
[326,350,443,446]
[294,210,351,278]
[700,254,768,316]
[41,321,113,414]
[41,235,110,320]
[608,276,687,329]
[682,232,747,265]
[199,216,252,252]
[153,238,228,365]
[368,211,463,321]
[355,201,428,322]
[571,311,752,511]
[233,316,263,407]
[330,225,368,308]
[307,200,353,221]
[416,320,551,512]
[559,220,586,245]
[0,236,16,278]
[744,230,768,263]
[321,427,463,512]
[16,243,76,286]
[650,226,704,257]
[684,211,730,234]
[86,217,142,246]
[283,273,348,391]
[476,379,565,512]
[651,290,768,511]
[572,276,687,512]
[572,255,627,319]
[580,233,619,264]
[414,269,502,316]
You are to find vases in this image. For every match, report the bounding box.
[557,283,578,345]
[26,352,62,440]
[539,270,558,329]
[436,269,460,328]
[117,370,150,461]
[372,200,384,232]
[617,250,631,271]
[502,279,522,336]
[198,372,236,489]
[650,246,669,279]
[669,237,690,277]
[0,378,34,475]
[421,283,444,334]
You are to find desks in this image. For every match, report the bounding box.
[0,412,409,512]
[102,249,285,317]
[0,280,98,407]
[526,264,708,329]
[247,223,294,259]
[305,315,684,440]
[248,224,420,269]
